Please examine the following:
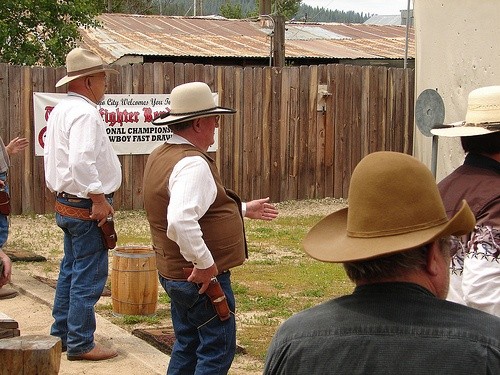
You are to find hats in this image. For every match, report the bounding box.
[303,151,476,263]
[153,81,237,126]
[431,85,500,137]
[55,48,119,88]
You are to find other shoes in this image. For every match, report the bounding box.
[0,283,19,299]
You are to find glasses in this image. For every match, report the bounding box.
[440,235,463,257]
[85,74,107,80]
[205,115,219,122]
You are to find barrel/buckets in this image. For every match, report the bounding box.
[111,246,158,317]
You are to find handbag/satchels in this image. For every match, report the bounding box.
[0,188,11,214]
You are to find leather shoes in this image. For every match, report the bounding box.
[67,345,118,361]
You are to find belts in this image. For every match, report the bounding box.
[56,191,115,198]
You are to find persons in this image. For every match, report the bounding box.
[44,48,122,361]
[430,85,500,318]
[262,152,500,375]
[143,82,279,375]
[0,135,29,300]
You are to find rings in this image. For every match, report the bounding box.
[106,216,112,221]
[210,277,218,284]
